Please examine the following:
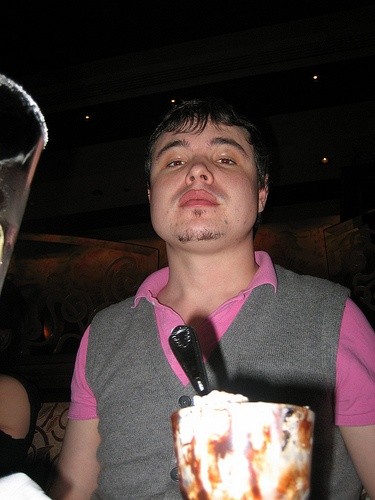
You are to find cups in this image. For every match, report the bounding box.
[171,403,314,500]
[0,75,49,293]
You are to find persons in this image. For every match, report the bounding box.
[43,96,375,500]
[0,279,40,478]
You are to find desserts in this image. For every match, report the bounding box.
[171,389,315,500]
